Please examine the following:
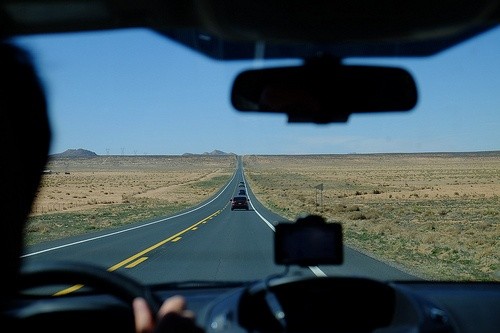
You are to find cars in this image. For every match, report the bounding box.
[229,195,250,211]
[238,181,246,195]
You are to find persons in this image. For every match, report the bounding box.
[0,40,206,333]
